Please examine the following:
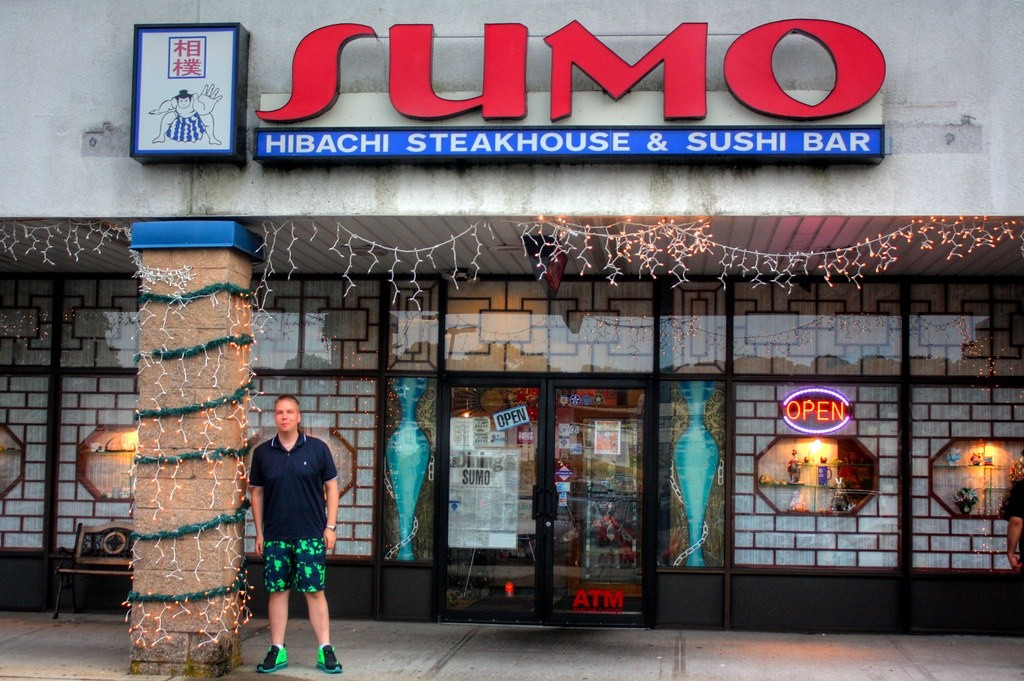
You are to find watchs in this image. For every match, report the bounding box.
[326,524,337,531]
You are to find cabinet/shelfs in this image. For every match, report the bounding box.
[758,458,874,496]
[932,464,1011,492]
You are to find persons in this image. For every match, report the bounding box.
[1003,448,1024,584]
[247,393,343,674]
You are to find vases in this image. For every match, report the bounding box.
[960,503,971,514]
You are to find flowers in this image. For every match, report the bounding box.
[952,486,979,507]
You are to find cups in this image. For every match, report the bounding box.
[818,466,832,485]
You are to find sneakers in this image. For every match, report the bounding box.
[257,644,288,672]
[316,645,343,673]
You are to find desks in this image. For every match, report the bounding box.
[568,496,641,579]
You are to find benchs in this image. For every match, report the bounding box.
[52,521,135,620]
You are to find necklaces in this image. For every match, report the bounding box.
[285,441,295,449]
